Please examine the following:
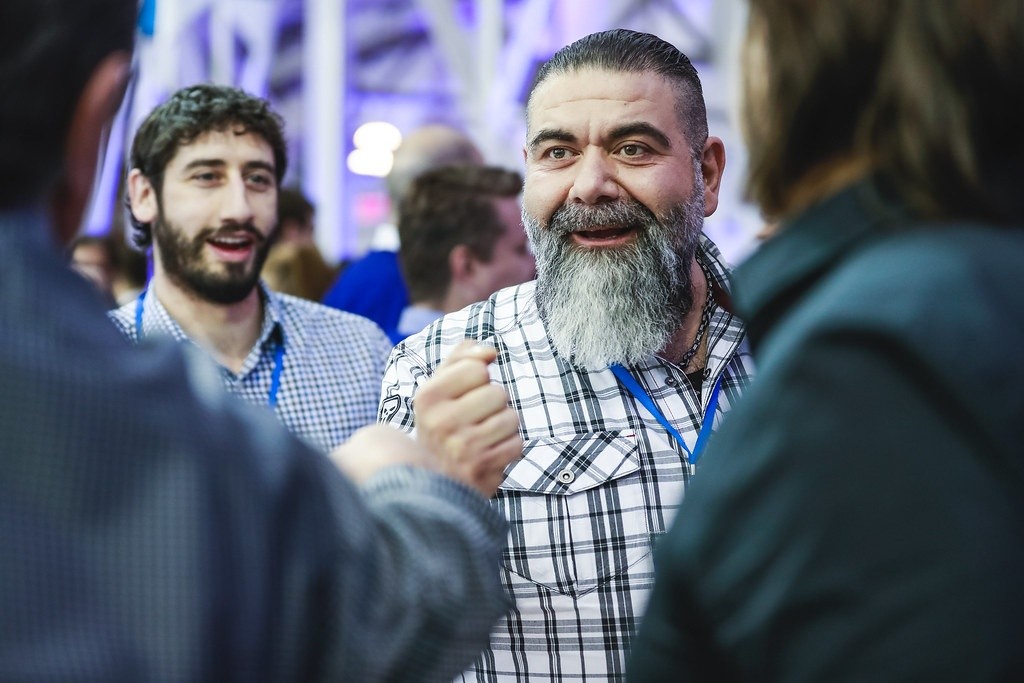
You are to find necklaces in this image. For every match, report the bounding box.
[601,359,721,478]
[675,258,715,371]
[134,289,286,411]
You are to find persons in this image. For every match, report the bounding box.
[320,30,755,683]
[392,162,540,350]
[322,121,483,346]
[64,178,342,313]
[103,85,395,454]
[630,1,1023,683]
[0,0,518,683]
